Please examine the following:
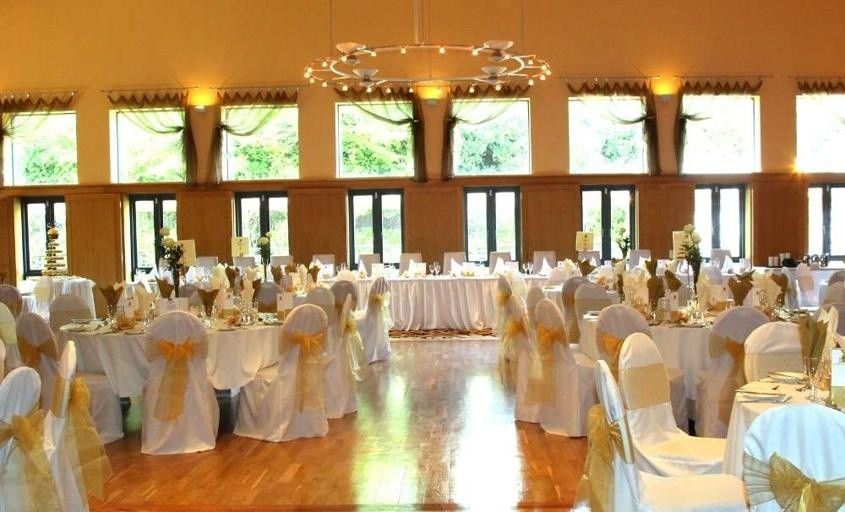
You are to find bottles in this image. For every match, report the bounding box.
[769,252,829,268]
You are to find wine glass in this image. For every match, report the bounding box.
[523,264,529,275]
[804,354,825,401]
[528,262,535,274]
[334,261,441,279]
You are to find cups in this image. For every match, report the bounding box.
[604,260,612,266]
[105,301,261,331]
[135,267,145,276]
[637,290,784,328]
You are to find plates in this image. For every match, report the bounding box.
[59,318,104,331]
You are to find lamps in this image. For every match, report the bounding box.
[181,87,220,113]
[303,1,554,110]
[643,75,687,103]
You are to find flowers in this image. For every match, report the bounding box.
[155,227,188,297]
[612,225,630,260]
[256,231,272,278]
[675,223,704,295]
